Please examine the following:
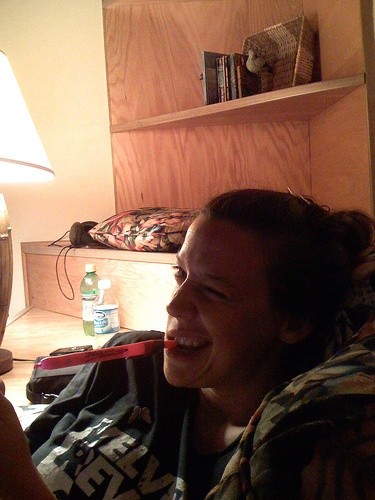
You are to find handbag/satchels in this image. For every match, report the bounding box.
[27,355,86,404]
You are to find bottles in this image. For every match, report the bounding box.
[79,263,102,337]
[90,279,121,347]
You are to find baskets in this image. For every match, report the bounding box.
[242,16,316,97]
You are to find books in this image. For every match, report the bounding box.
[201,51,257,106]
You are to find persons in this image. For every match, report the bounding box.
[0,189,374,500]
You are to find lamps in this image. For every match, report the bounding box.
[0,50,55,375]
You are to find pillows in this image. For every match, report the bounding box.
[89,207,201,253]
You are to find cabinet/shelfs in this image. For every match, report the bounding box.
[20,0,375,332]
